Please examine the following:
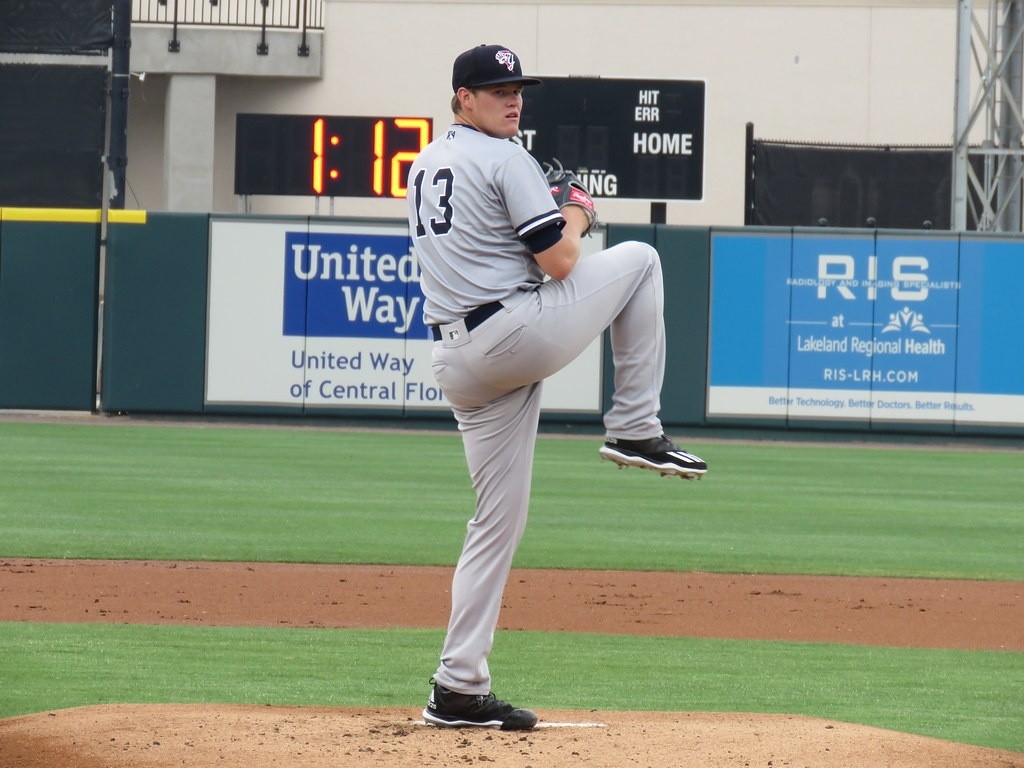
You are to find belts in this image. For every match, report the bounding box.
[431,300,504,341]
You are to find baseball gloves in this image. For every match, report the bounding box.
[545,169,599,239]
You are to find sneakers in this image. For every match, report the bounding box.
[599,434,708,479]
[422,676,538,731]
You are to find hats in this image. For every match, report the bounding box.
[452,44,542,93]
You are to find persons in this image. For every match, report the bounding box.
[404,44,710,729]
